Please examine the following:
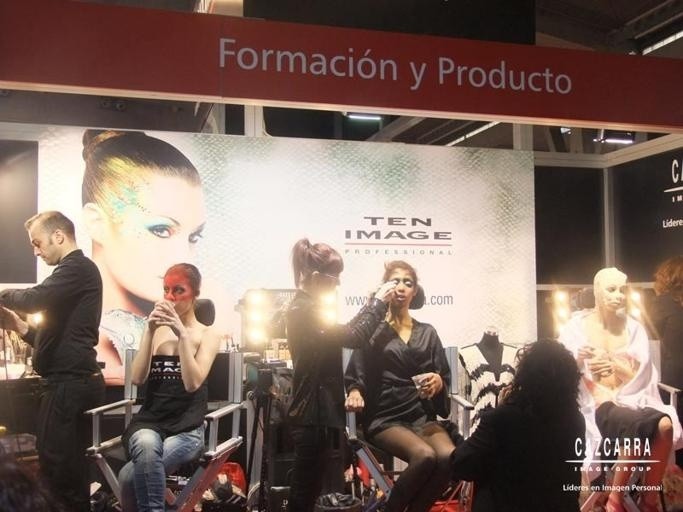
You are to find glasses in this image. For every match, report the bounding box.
[324,274,338,279]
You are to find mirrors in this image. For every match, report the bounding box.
[0,288,36,366]
[536,287,663,355]
[240,287,302,372]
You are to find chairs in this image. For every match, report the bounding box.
[85,350,244,511]
[651,340,681,412]
[343,346,475,512]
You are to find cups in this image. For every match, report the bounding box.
[583,346,597,373]
[411,375,430,399]
[154,301,174,318]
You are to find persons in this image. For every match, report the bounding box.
[286,238,396,512]
[644,256,683,469]
[558,267,673,512]
[449,338,586,512]
[82,129,207,386]
[118,263,220,512]
[344,261,456,512]
[458,326,524,437]
[0,211,107,512]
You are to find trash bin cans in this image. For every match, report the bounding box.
[316,493,363,512]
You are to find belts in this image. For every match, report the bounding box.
[48,362,105,382]
